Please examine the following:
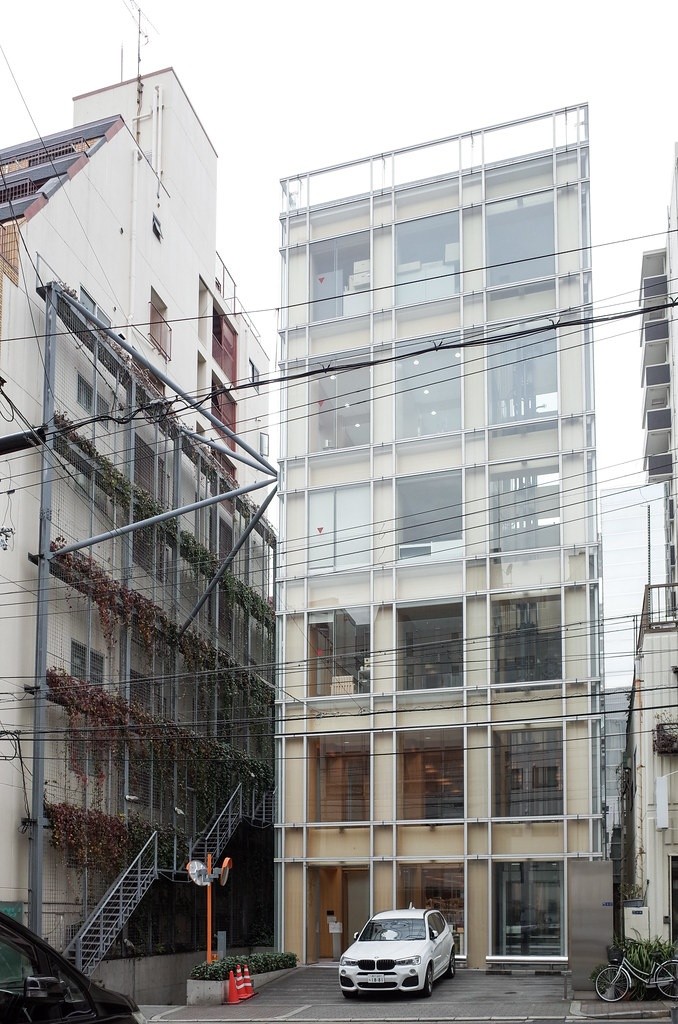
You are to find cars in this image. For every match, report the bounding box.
[0,912,149,1023]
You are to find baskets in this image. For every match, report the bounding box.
[607,946,621,964]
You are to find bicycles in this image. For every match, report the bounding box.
[594,944,678,1003]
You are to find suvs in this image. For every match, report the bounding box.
[339,907,457,998]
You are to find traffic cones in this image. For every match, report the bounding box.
[235,964,252,1000]
[222,971,244,1005]
[243,964,259,996]
